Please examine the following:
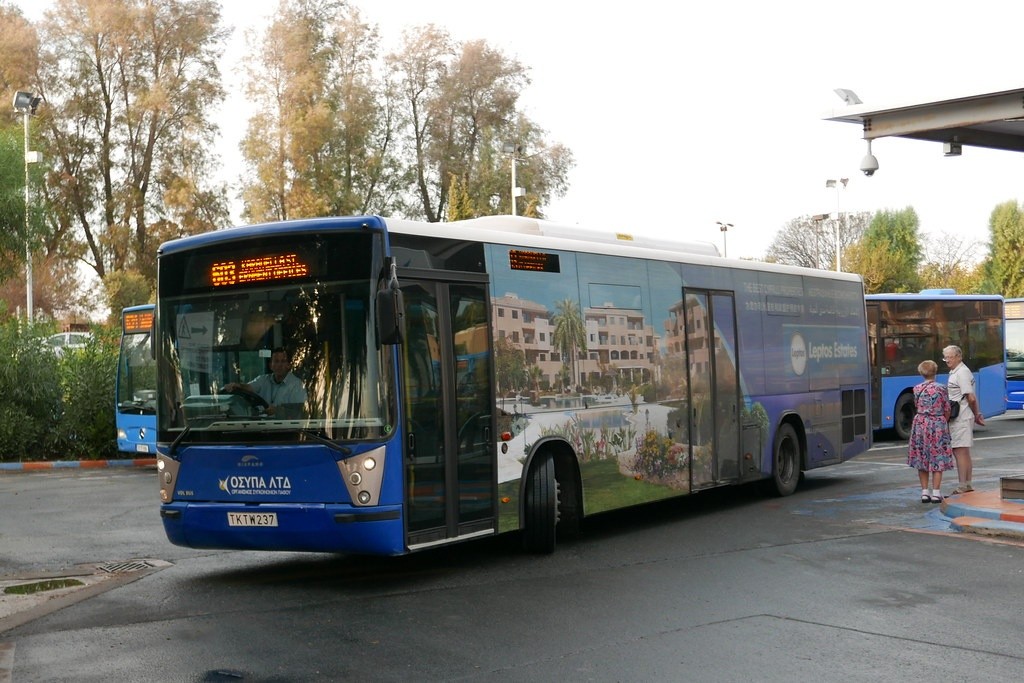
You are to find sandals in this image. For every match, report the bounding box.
[931,493,943,503]
[921,495,931,503]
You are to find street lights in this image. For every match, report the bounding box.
[826,176,851,272]
[717,221,734,259]
[12,89,42,322]
[502,143,525,216]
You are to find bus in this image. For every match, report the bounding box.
[149,214,874,555]
[115,304,155,455]
[1001,298,1024,411]
[861,289,1009,441]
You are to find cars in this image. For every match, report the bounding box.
[39,331,98,360]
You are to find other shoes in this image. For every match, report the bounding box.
[944,483,974,498]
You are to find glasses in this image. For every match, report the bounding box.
[942,355,957,362]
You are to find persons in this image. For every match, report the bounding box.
[220,348,308,410]
[907,361,952,503]
[942,346,986,498]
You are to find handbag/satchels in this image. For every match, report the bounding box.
[949,400,960,418]
